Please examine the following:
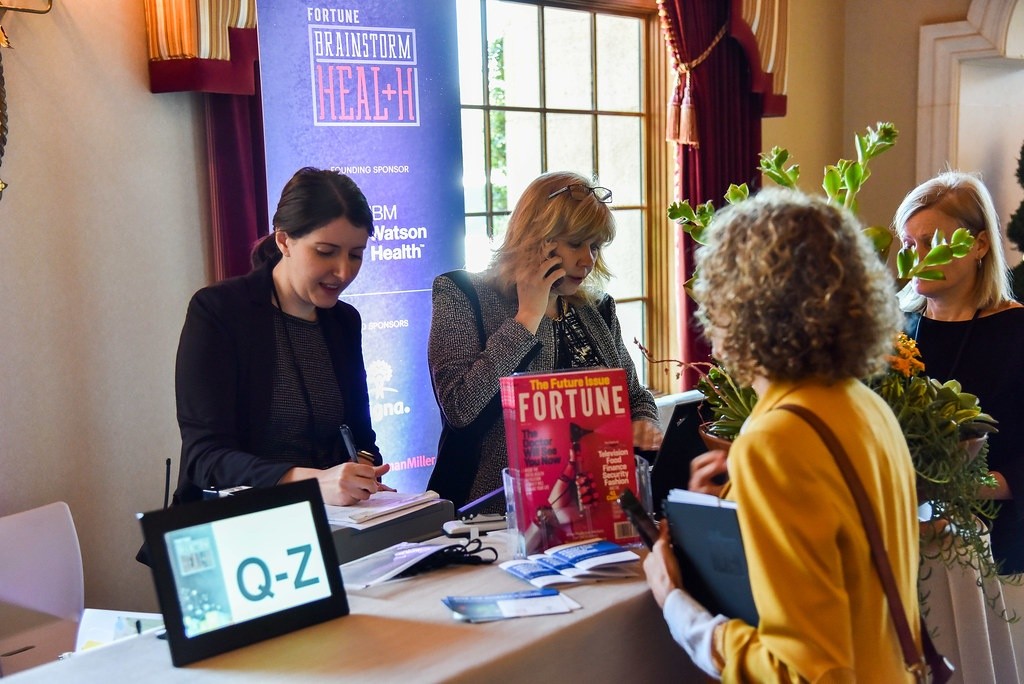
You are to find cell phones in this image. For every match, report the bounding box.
[616,489,660,553]
[543,251,565,291]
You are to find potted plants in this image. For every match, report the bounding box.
[632,118,1024,625]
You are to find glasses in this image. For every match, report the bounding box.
[548,184,613,203]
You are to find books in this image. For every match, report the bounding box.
[497,536,642,592]
[498,368,642,558]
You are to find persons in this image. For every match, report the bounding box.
[169,167,397,567]
[426,172,664,514]
[641,185,929,684]
[888,160,1024,576]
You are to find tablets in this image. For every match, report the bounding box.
[137,477,350,667]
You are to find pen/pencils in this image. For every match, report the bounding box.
[339,424,360,463]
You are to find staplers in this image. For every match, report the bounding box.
[441,486,508,538]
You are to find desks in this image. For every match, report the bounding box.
[0,528,707,683]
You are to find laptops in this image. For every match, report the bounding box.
[649,398,719,521]
